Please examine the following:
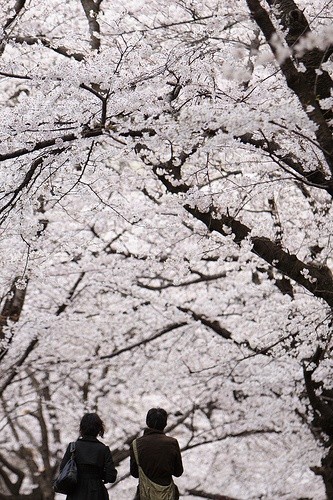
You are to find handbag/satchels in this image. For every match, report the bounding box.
[136,468,176,500]
[53,441,78,494]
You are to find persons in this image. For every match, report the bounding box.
[59,412,118,499]
[129,407,184,499]
